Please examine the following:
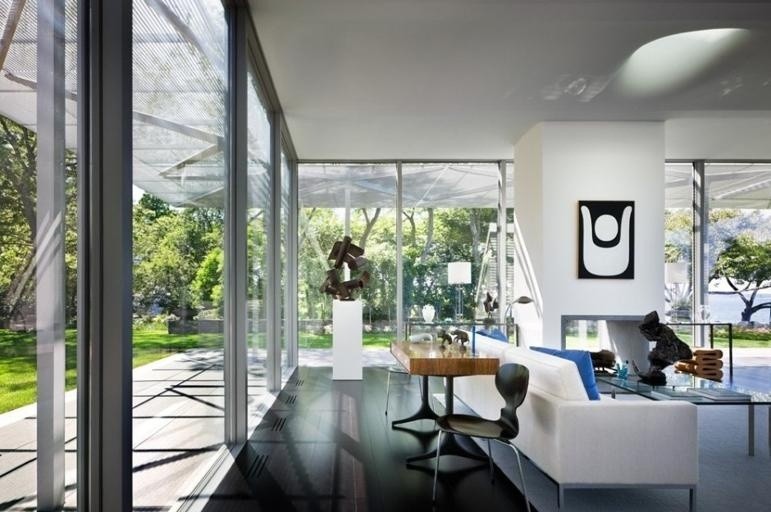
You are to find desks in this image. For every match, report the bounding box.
[404,315,519,346]
[665,321,736,386]
[390,339,500,465]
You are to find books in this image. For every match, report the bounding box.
[686,387,751,401]
[650,389,703,400]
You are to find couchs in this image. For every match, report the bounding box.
[443,333,699,511]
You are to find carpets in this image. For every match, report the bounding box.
[429,366,771,511]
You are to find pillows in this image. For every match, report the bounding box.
[531,344,602,400]
[475,326,509,342]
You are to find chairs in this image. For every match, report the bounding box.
[434,363,532,510]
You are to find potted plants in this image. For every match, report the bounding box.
[483,291,499,329]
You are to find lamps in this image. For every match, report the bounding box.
[422,304,436,324]
[447,262,474,317]
